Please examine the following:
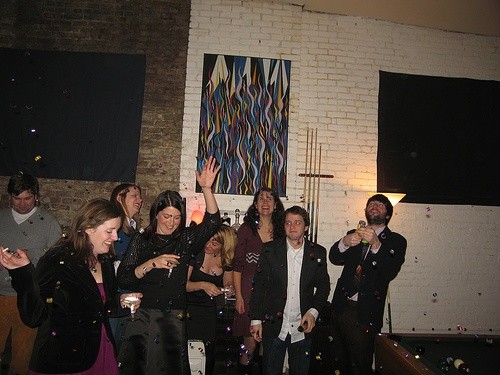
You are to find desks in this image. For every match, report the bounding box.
[375,332,500,375]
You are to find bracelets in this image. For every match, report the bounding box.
[200,186,211,189]
[343,235,350,246]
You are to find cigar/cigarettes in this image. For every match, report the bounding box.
[2,247,8,252]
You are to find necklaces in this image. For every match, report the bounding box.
[91,267,97,273]
[158,235,174,243]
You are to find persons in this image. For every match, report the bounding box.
[0,156,331,375]
[329,194,407,375]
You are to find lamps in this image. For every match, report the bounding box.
[368,192,406,207]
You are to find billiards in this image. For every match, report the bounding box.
[434,337,440,343]
[416,345,425,354]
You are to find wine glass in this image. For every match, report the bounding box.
[164,252,181,278]
[120,292,140,322]
[220,285,231,304]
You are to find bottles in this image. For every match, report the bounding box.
[221,212,231,227]
[231,213,241,235]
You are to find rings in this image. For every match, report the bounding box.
[166,261,169,266]
[211,292,214,295]
[252,331,256,334]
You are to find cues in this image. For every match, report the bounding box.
[310,128,317,242]
[299,127,309,209]
[306,127,313,239]
[387,284,392,333]
[314,143,321,243]
